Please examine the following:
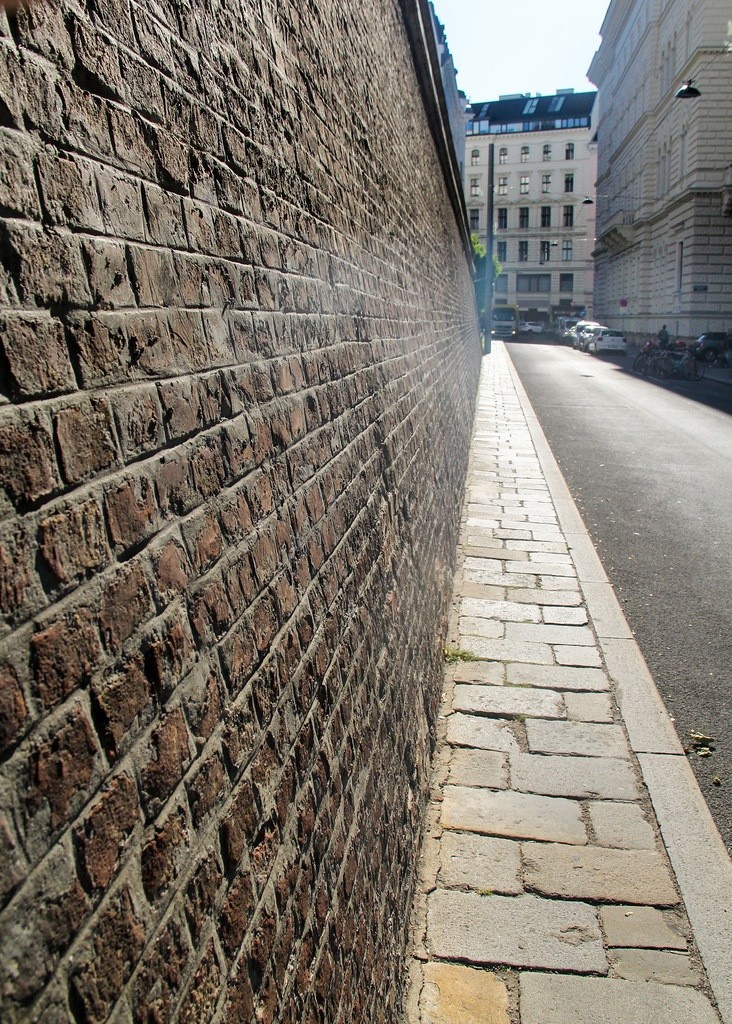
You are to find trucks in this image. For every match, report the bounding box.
[489,304,520,338]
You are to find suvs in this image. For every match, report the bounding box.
[518,321,546,336]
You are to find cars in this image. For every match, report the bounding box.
[546,317,627,357]
[691,331,732,362]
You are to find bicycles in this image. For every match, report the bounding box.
[633,341,706,381]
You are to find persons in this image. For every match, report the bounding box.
[656,324,670,346]
